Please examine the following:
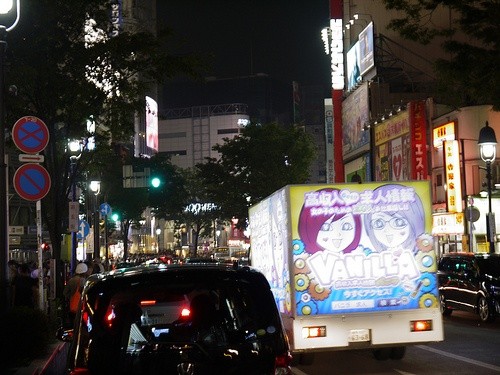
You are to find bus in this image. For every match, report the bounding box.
[213,247,230,261]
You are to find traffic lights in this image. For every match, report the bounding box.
[150,177,177,188]
[112,213,119,222]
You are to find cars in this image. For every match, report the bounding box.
[55,253,293,375]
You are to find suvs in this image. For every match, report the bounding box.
[439,251,500,324]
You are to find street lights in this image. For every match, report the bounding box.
[156,222,161,255]
[243,230,248,245]
[216,229,220,248]
[66,136,84,277]
[477,121,498,252]
[88,179,102,259]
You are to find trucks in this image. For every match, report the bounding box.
[241,180,444,365]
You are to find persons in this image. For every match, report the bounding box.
[7,258,50,313]
[84,257,104,275]
[62,262,89,328]
